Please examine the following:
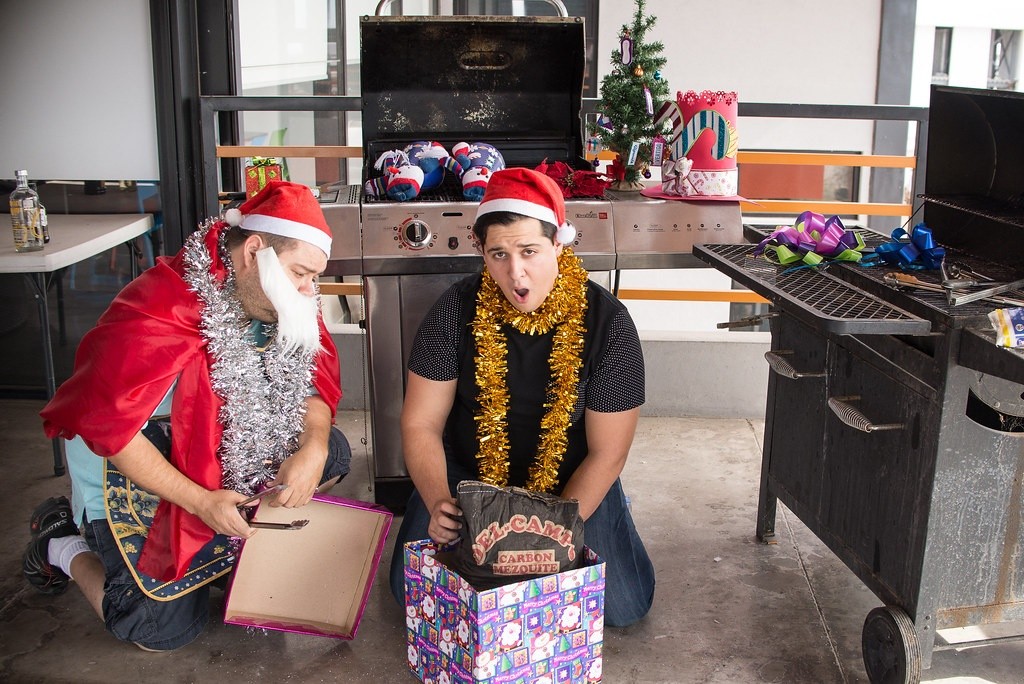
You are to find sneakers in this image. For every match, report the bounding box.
[22,496,81,596]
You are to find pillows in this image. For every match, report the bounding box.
[440,480,584,592]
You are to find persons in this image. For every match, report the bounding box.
[388,166,655,629]
[22,181,352,653]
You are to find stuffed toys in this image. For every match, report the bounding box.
[365,141,506,202]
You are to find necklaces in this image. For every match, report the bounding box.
[184,215,316,513]
[472,245,590,492]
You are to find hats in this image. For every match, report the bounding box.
[226,181,334,260]
[473,168,577,244]
[640,89,766,208]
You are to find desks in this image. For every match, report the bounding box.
[0,213,155,478]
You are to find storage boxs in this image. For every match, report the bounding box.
[405,537,606,684]
[244,155,283,201]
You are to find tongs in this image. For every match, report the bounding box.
[884,262,1024,306]
[941,262,1024,306]
[236,484,309,531]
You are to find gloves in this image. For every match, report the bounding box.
[364,140,447,200]
[437,141,505,199]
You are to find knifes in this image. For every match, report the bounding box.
[941,280,1019,290]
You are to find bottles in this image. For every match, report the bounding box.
[28,183,50,243]
[10,169,44,252]
